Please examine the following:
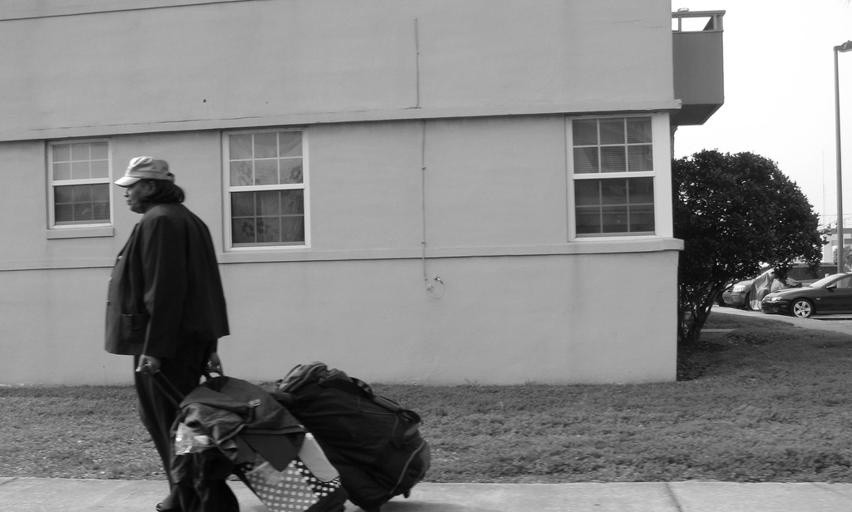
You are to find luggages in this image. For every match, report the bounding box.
[273,363,431,512]
[180,367,348,512]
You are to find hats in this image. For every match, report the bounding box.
[113,156,175,186]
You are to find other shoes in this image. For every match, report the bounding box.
[156,496,171,512]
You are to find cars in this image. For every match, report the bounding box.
[722,262,852,319]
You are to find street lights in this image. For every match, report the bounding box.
[832,40,852,288]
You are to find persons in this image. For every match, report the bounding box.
[103,156,232,511]
